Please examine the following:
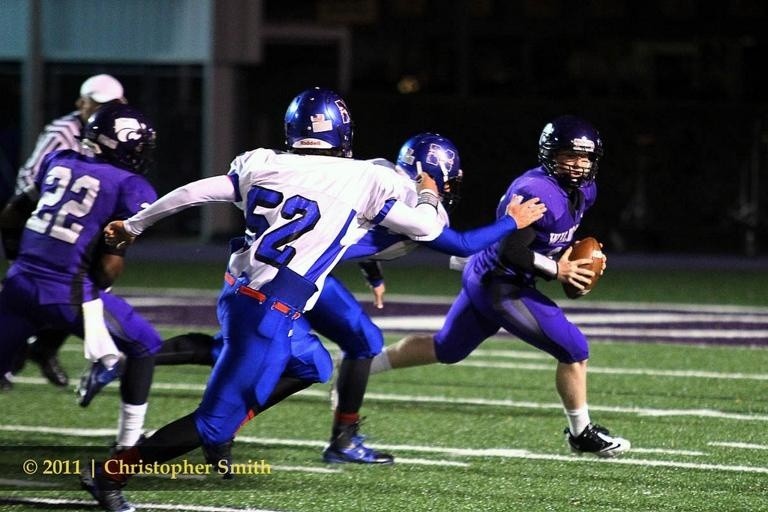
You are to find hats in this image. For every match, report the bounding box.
[78,73,127,105]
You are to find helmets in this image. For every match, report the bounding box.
[82,108,159,173]
[394,131,463,210]
[283,86,358,158]
[536,114,603,190]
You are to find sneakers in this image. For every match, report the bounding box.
[77,462,138,512]
[38,351,71,390]
[564,423,630,459]
[1,373,14,392]
[75,352,122,410]
[320,437,396,467]
[329,349,344,414]
[201,436,235,479]
[109,435,146,454]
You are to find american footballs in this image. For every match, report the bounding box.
[563,237,603,298]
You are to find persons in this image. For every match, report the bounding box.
[80,133,465,465]
[322,119,630,457]
[15,74,126,383]
[1,103,163,451]
[78,84,546,512]
